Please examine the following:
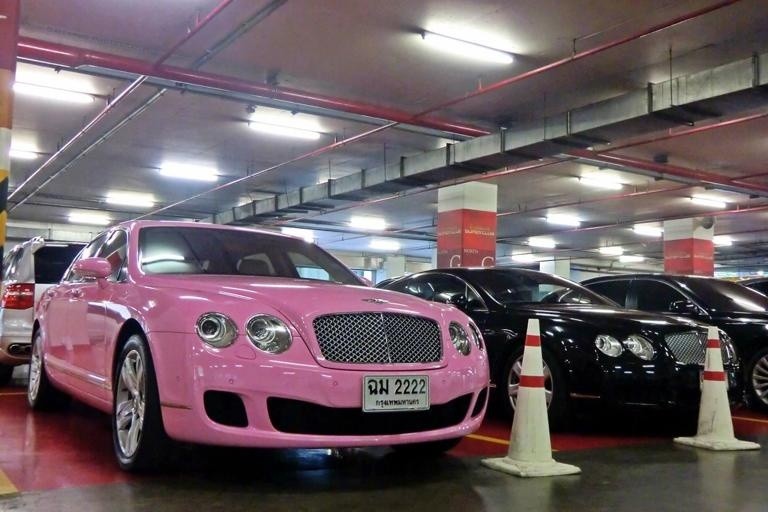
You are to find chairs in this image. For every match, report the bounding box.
[238,258,270,275]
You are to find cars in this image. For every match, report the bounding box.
[27,219,491,472]
[540,275,768,418]
[377,267,738,422]
[0,237,89,386]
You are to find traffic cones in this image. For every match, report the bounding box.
[674,325,760,451]
[482,318,581,479]
